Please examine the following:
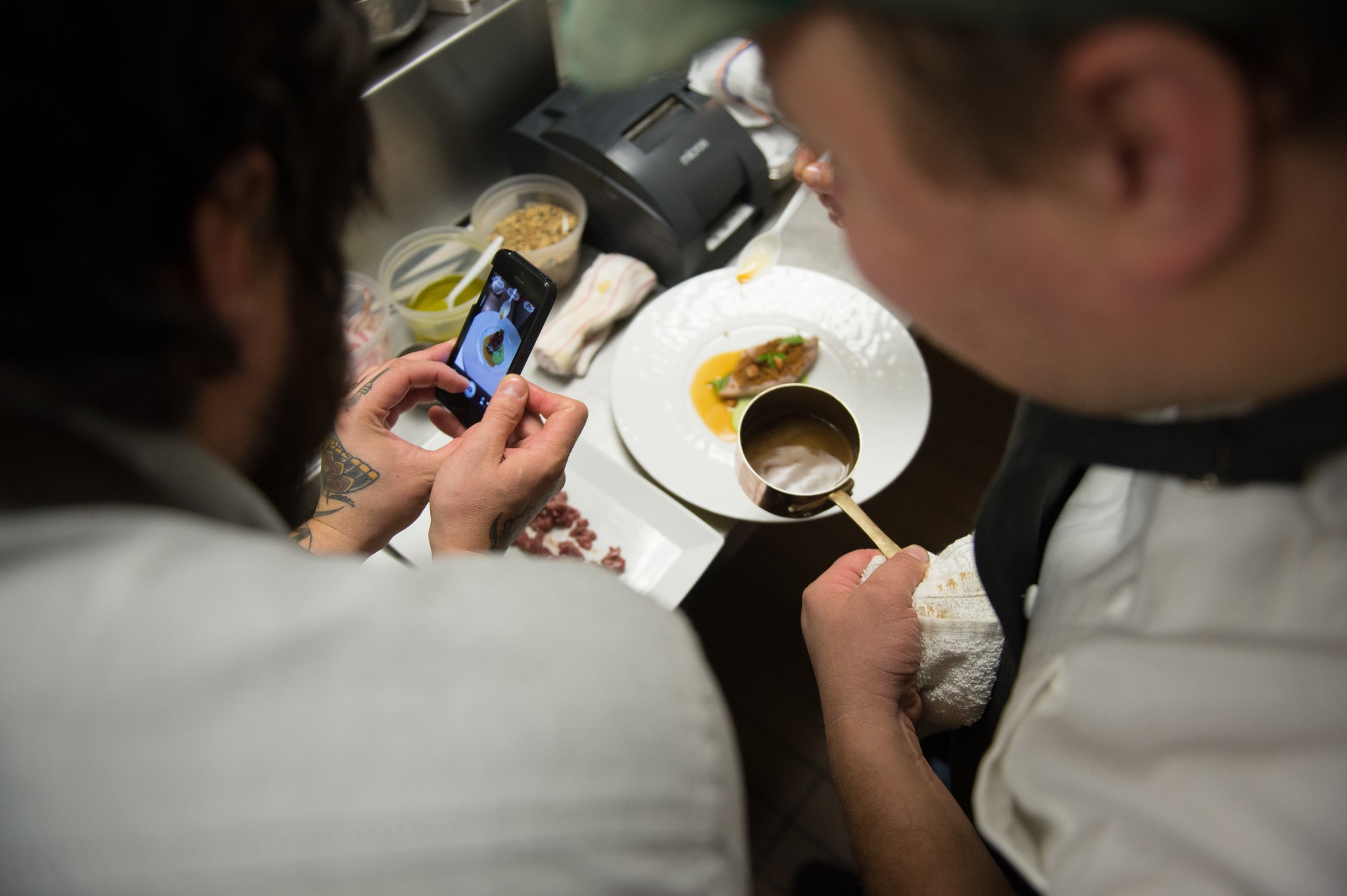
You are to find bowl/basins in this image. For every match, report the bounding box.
[470,173,588,288]
[379,227,501,344]
[345,270,392,388]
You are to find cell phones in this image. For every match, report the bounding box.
[435,249,559,429]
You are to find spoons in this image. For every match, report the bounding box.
[500,287,517,318]
[443,233,505,309]
[735,150,831,284]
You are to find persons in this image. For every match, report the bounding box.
[757,1,1347,893]
[1,1,755,896]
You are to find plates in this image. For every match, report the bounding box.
[610,265,932,523]
[462,309,521,399]
[377,331,740,616]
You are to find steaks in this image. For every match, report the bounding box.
[716,334,819,399]
[486,331,504,353]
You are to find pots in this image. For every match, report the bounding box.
[736,384,903,561]
